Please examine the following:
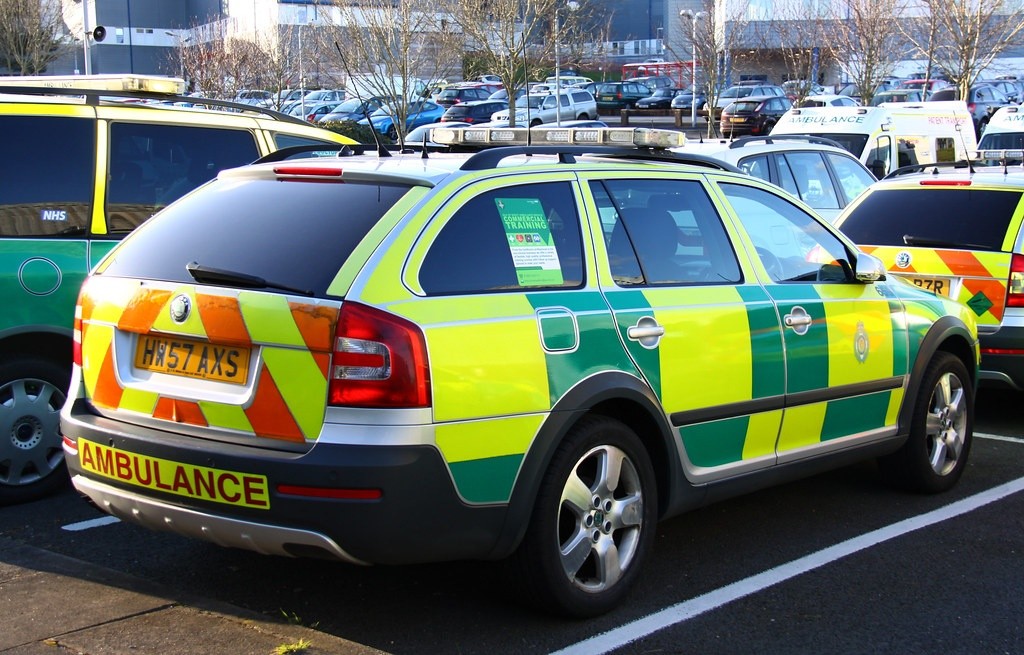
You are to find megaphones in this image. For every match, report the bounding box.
[92,26,106,42]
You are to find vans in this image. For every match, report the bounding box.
[545,75,593,84]
[768,99,981,182]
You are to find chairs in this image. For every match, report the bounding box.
[608,208,681,281]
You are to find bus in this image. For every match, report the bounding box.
[622,59,712,90]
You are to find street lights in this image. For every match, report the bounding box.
[555,2,581,127]
[692,11,710,127]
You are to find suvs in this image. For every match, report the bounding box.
[491,90,599,127]
[596,80,655,116]
[597,130,880,270]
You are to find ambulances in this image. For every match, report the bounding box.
[59,125,980,623]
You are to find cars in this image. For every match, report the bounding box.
[634,87,685,110]
[869,87,932,107]
[530,119,606,128]
[838,71,1024,101]
[581,82,605,101]
[927,84,1020,144]
[0,84,366,504]
[802,150,1024,395]
[670,82,722,112]
[781,80,826,104]
[976,106,1024,169]
[719,94,795,140]
[102,70,565,146]
[789,95,861,111]
[701,83,790,123]
